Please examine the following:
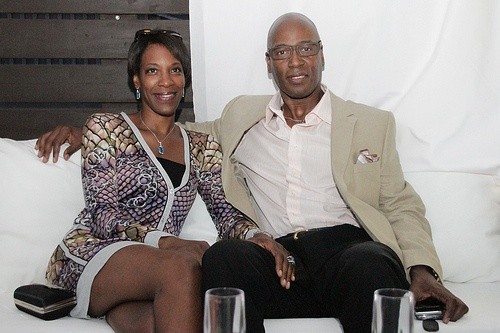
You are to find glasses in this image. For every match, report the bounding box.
[266,40,322,60]
[134,29,183,44]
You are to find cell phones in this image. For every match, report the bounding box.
[414,298,445,321]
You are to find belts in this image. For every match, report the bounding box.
[285,228,328,240]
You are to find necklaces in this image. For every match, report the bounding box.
[285,117,305,123]
[140,110,174,154]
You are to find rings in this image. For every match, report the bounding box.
[287,256,295,264]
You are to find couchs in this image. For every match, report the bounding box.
[0,136,500,333]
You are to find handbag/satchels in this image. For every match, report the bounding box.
[14,284,77,321]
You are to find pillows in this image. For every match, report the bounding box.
[400,171,500,283]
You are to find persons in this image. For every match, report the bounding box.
[44,29,299,333]
[35,12,469,333]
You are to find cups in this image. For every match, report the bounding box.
[203,287,246,333]
[371,289,414,333]
